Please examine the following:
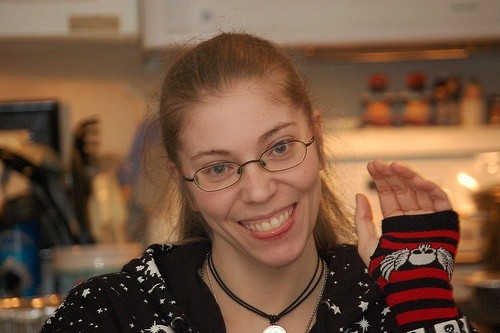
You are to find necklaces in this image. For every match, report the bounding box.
[201,250,328,333]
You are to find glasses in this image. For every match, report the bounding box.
[177,136,315,192]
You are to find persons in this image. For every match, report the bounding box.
[39,31,481,333]
